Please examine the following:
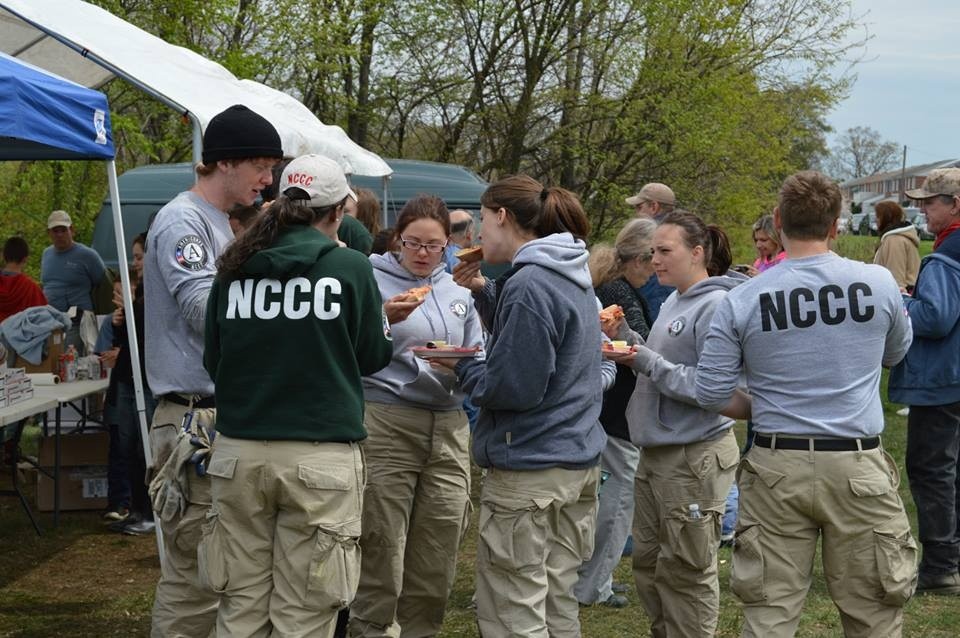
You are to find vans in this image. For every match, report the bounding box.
[91,159,495,271]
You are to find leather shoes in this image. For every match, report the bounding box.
[122,520,155,536]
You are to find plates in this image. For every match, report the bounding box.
[407,346,476,358]
[601,348,632,357]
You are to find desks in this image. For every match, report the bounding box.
[0,378,110,537]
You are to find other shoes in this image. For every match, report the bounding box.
[611,583,632,594]
[916,563,960,596]
[579,595,628,609]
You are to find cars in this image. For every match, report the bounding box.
[836,207,937,241]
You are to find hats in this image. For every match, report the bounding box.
[48,210,72,229]
[625,183,676,207]
[201,104,283,165]
[905,167,960,200]
[279,154,358,208]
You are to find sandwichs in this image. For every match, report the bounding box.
[599,304,634,351]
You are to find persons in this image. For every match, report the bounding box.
[574,179,786,608]
[338,187,484,475]
[202,152,393,638]
[598,211,752,638]
[425,175,603,638]
[698,171,913,638]
[145,105,281,638]
[41,210,113,363]
[0,236,49,471]
[93,232,159,536]
[346,196,488,638]
[889,166,960,594]
[872,201,921,416]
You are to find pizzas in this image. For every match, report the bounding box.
[455,246,483,262]
[398,285,433,302]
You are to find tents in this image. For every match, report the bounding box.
[0,52,166,575]
[0,0,394,232]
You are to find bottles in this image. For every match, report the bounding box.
[55,345,108,383]
[686,503,704,519]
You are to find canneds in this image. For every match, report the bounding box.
[58,353,75,381]
[97,357,107,379]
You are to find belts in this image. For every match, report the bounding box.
[755,432,880,451]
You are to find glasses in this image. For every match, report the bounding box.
[400,233,449,253]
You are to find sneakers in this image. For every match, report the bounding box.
[103,508,129,519]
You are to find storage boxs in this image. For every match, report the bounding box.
[16,328,65,374]
[36,433,110,510]
